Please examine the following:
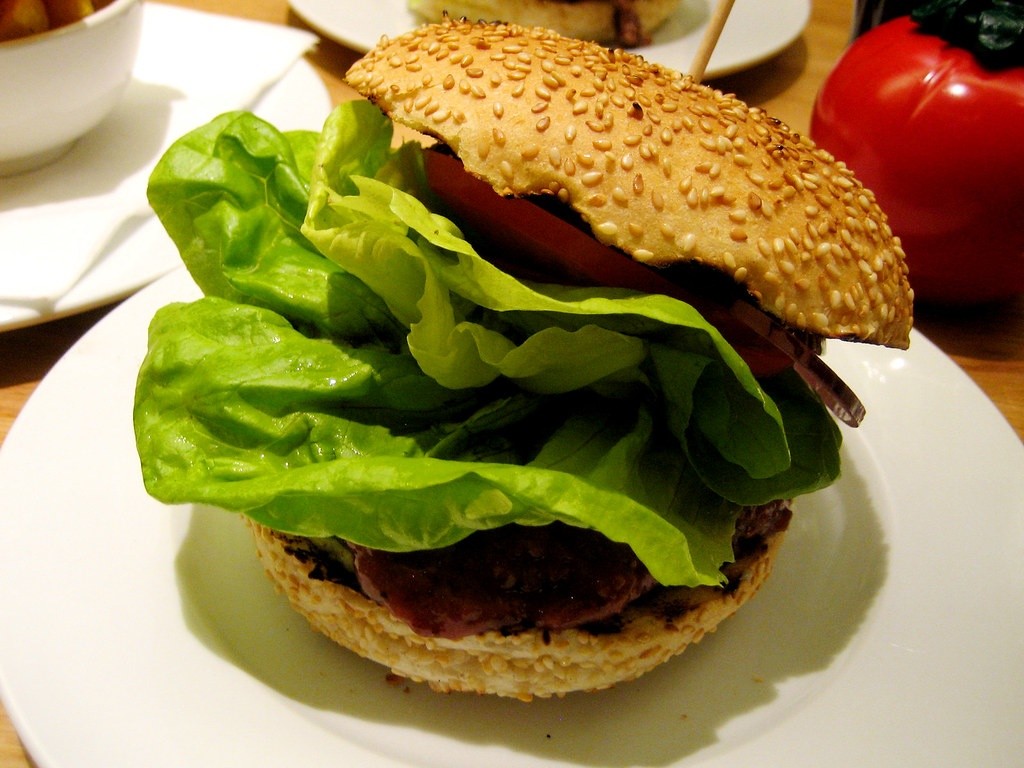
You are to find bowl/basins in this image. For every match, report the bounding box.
[0,0,143,177]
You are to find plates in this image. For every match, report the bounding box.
[0,0,332,336]
[0,261,1024,768]
[289,0,812,80]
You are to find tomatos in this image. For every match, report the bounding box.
[804,0,1024,317]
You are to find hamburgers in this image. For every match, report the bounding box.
[132,12,913,702]
